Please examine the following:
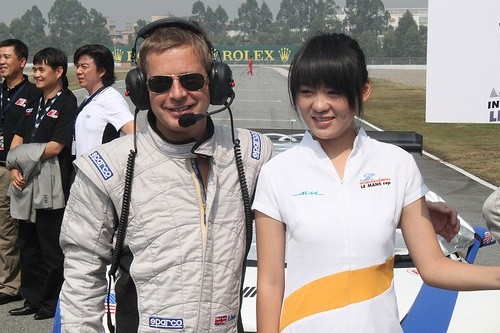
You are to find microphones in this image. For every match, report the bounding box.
[179,89,236,128]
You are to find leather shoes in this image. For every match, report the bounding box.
[9,303,39,314]
[34,308,54,319]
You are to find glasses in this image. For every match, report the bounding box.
[146,72,208,94]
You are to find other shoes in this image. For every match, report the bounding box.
[0,292,25,305]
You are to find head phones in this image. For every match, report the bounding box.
[125,16,235,111]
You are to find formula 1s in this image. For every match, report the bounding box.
[53,128,500,333]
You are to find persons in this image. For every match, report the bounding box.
[0,38,140,319]
[247,56,253,75]
[59,17,460,333]
[251,33,500,333]
